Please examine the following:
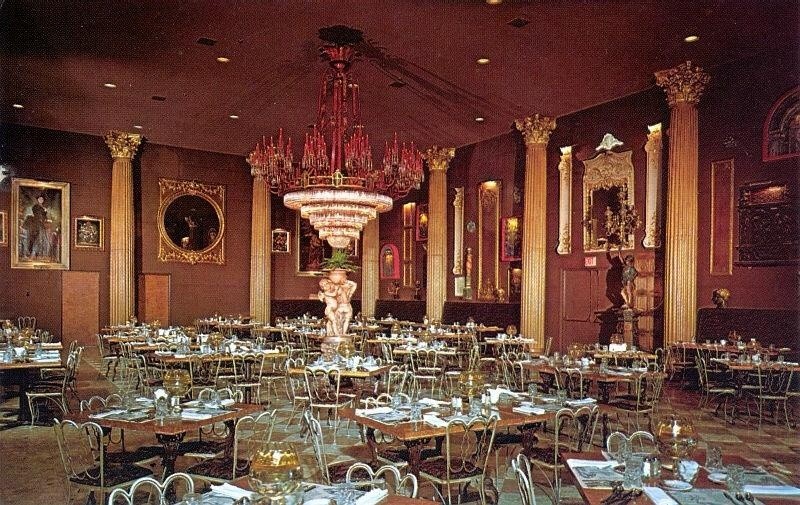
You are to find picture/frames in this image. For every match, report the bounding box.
[271,227,291,254]
[10,178,71,272]
[74,215,105,252]
[415,205,428,240]
[501,216,523,263]
[294,210,360,278]
[0,209,9,248]
[157,177,227,266]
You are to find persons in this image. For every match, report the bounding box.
[618,245,638,310]
[712,288,731,308]
[27,196,57,262]
[317,269,357,336]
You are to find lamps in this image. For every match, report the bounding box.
[603,180,643,249]
[245,25,425,250]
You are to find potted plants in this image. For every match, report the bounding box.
[317,248,362,274]
